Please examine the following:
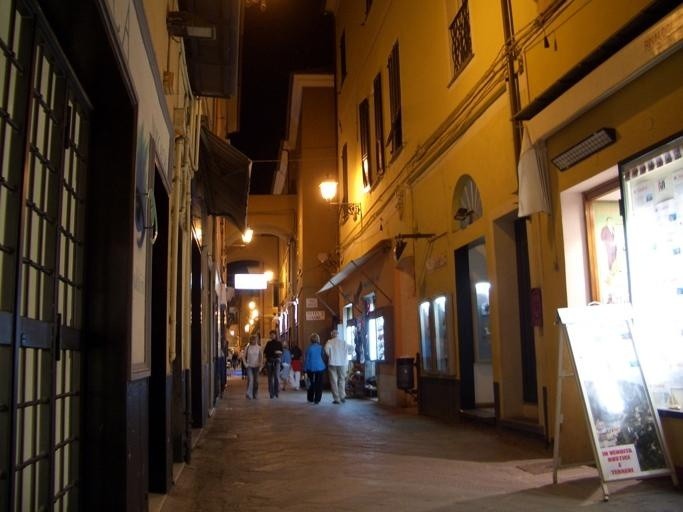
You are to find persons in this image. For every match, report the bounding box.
[232,329,348,405]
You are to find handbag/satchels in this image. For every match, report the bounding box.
[300,377,310,390]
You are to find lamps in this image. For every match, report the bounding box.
[318,170,362,221]
[166,11,216,40]
